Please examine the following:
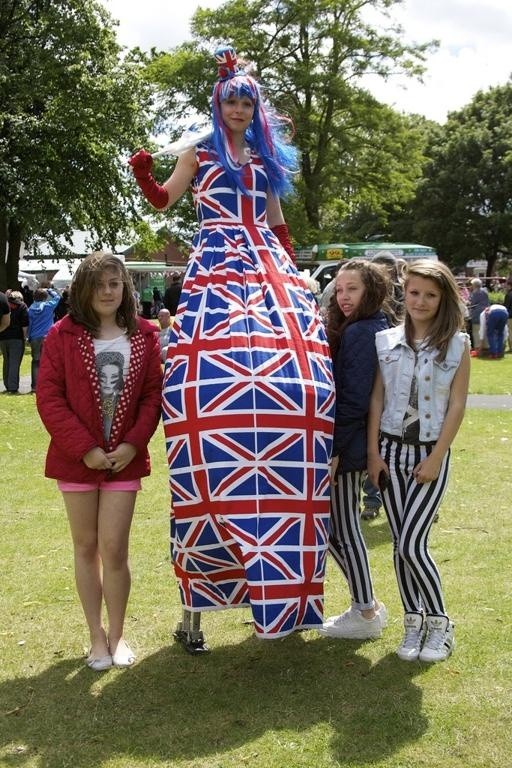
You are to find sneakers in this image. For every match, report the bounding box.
[317,609,383,639]
[418,615,455,663]
[326,602,389,629]
[398,611,429,661]
[87,645,112,671]
[361,506,379,520]
[111,639,136,668]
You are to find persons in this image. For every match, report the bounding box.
[319,260,388,639]
[127,45,339,639]
[36,252,159,672]
[366,258,473,664]
[1,275,183,395]
[446,243,511,360]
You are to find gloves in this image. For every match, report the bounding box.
[270,223,297,266]
[129,148,168,209]
